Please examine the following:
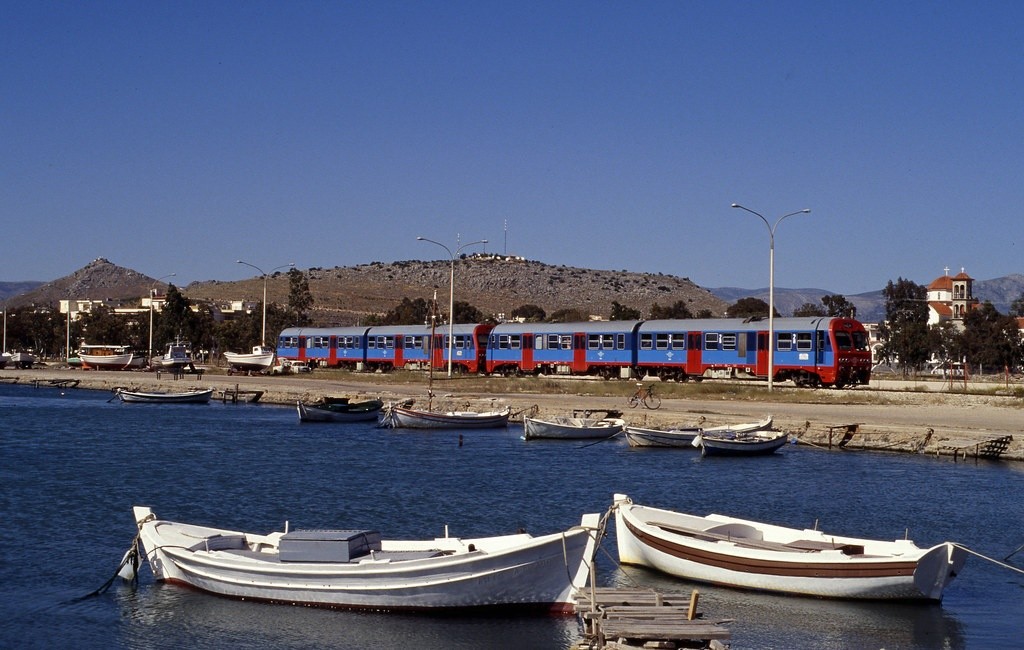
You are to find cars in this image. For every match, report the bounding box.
[273,360,310,374]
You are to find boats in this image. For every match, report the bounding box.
[10,352,38,363]
[612,492,971,602]
[148,334,193,370]
[622,414,792,457]
[521,415,627,442]
[133,504,590,611]
[382,397,512,430]
[296,396,384,423]
[111,386,216,403]
[222,351,275,372]
[75,344,133,370]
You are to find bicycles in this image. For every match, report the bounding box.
[624,383,662,410]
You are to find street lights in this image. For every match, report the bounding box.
[417,237,489,378]
[731,202,811,392]
[147,272,176,368]
[235,259,296,347]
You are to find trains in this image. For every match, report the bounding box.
[274,317,874,393]
